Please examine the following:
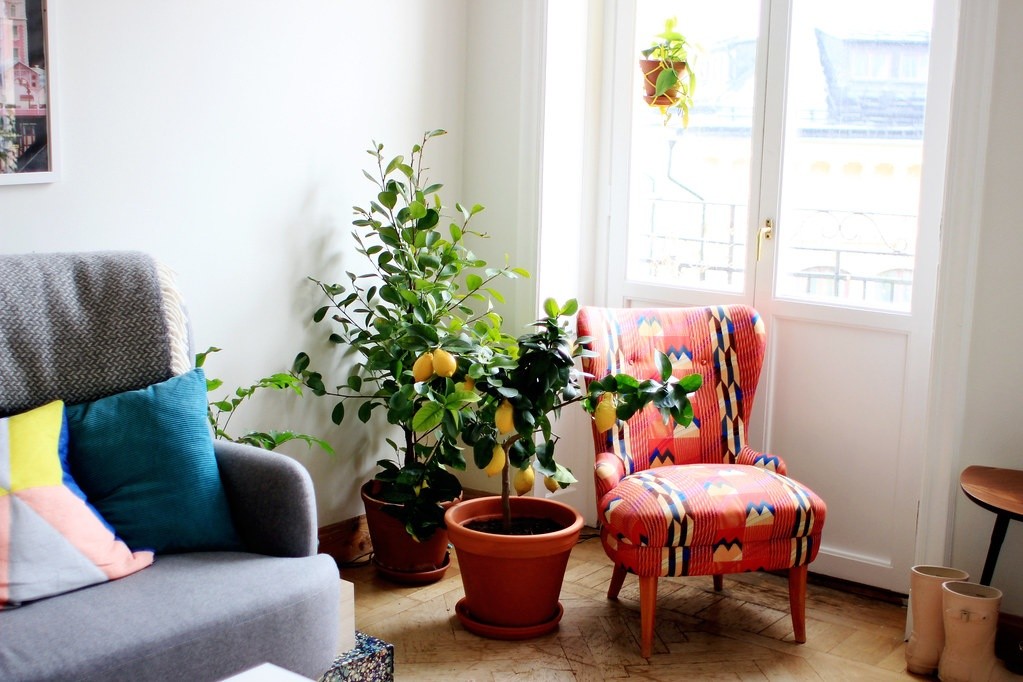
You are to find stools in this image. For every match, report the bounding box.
[960,466,1023,587]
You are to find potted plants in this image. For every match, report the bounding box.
[635,19,700,123]
[409,295,704,644]
[277,126,531,585]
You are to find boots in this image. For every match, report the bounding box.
[938,580,1023,682]
[904,565,970,674]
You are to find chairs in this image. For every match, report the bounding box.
[575,304,826,659]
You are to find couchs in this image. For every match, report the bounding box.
[0,252,357,682]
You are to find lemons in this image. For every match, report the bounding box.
[413,349,616,496]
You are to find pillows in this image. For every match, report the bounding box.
[0,397,158,611]
[64,367,235,553]
